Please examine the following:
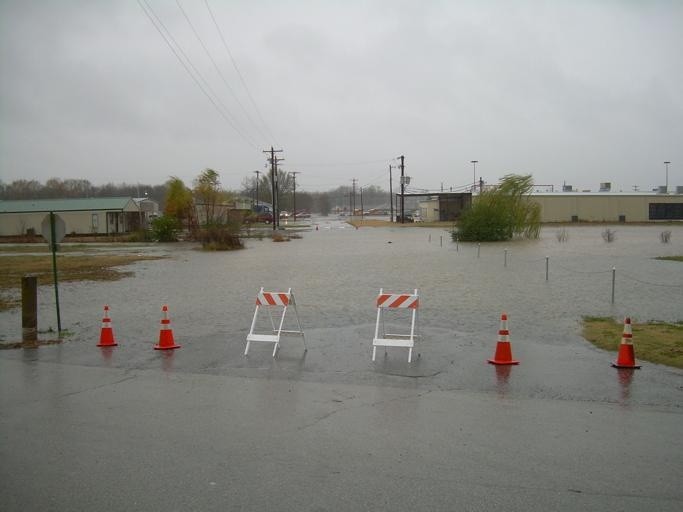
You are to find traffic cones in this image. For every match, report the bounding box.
[153,305,182,350]
[486,311,520,366]
[609,316,642,370]
[95,304,118,348]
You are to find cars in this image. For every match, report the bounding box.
[397,214,425,223]
[363,210,370,216]
[279,211,287,219]
[243,212,272,225]
[295,212,311,218]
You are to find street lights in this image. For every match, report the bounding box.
[470,160,478,186]
[289,171,301,217]
[664,161,671,187]
[253,170,261,206]
